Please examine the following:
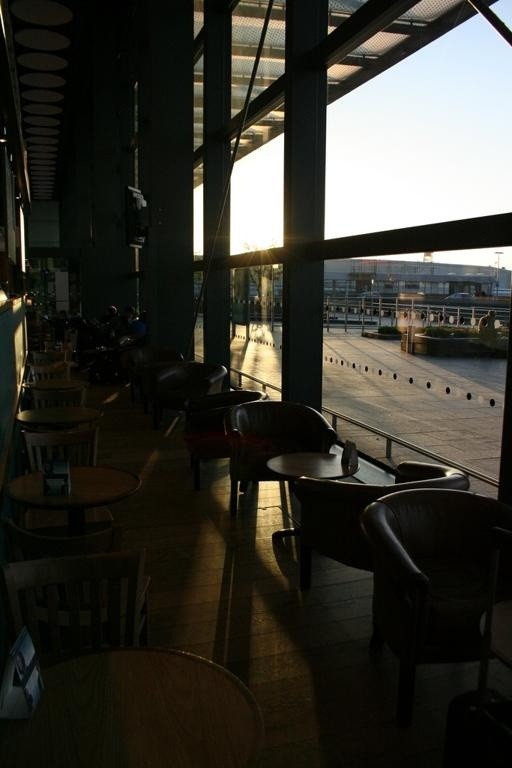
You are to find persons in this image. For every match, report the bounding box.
[478,310,501,359]
[49,301,140,383]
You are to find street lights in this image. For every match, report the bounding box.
[270,263,279,332]
[494,251,503,296]
[370,271,376,320]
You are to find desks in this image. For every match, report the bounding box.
[264,449,394,551]
[477,599,512,708]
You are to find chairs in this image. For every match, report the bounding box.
[359,486,510,728]
[231,394,339,518]
[2,350,230,766]
[290,460,470,594]
[229,384,512,768]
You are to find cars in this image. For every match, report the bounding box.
[355,288,428,304]
[471,290,492,297]
[440,292,483,307]
